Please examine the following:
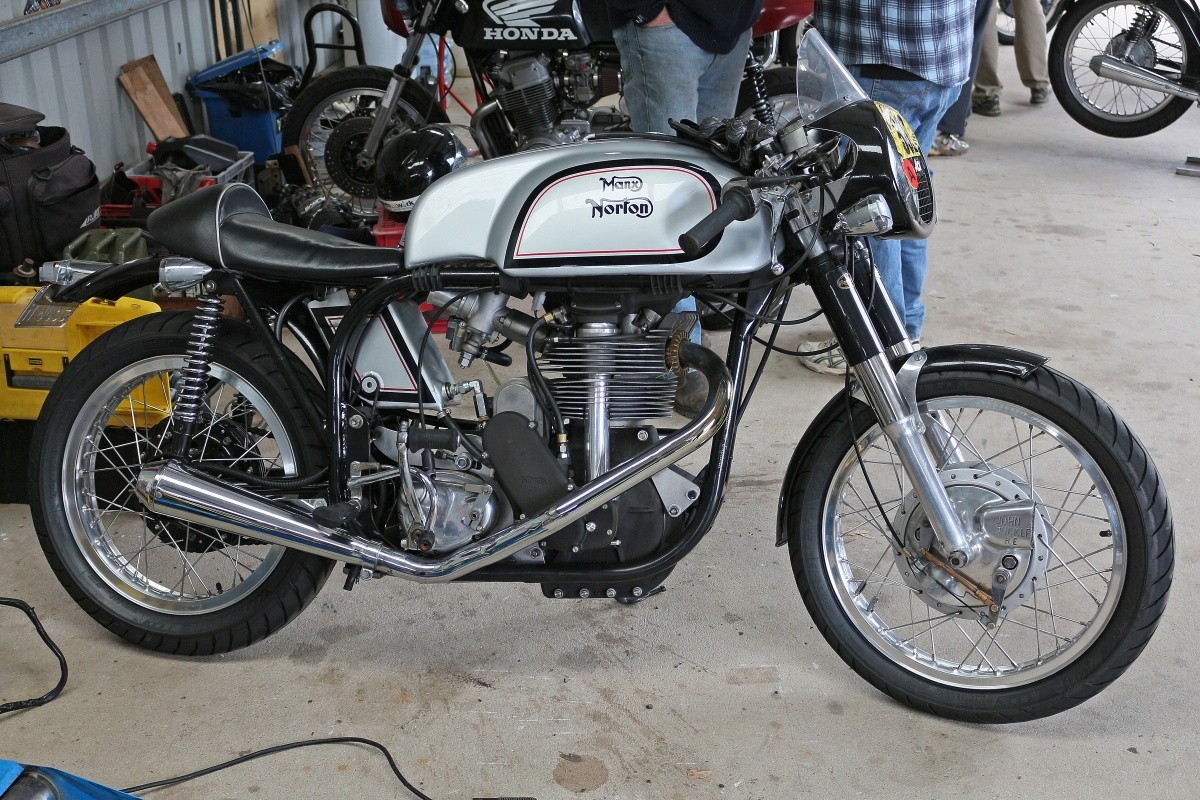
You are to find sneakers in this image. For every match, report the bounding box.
[798,340,854,376]
[970,94,1001,115]
[929,134,969,157]
[1031,89,1047,104]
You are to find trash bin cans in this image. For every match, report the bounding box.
[185,38,303,163]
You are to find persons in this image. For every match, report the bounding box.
[797,0,974,376]
[927,0,992,158]
[608,0,769,416]
[972,0,1051,115]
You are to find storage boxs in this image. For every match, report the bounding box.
[101,151,256,229]
[371,203,450,335]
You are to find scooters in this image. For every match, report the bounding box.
[1047,0,1200,177]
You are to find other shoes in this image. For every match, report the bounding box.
[674,372,709,416]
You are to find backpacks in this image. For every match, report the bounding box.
[0,91,103,269]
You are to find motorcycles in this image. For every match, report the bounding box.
[19,0,1186,726]
